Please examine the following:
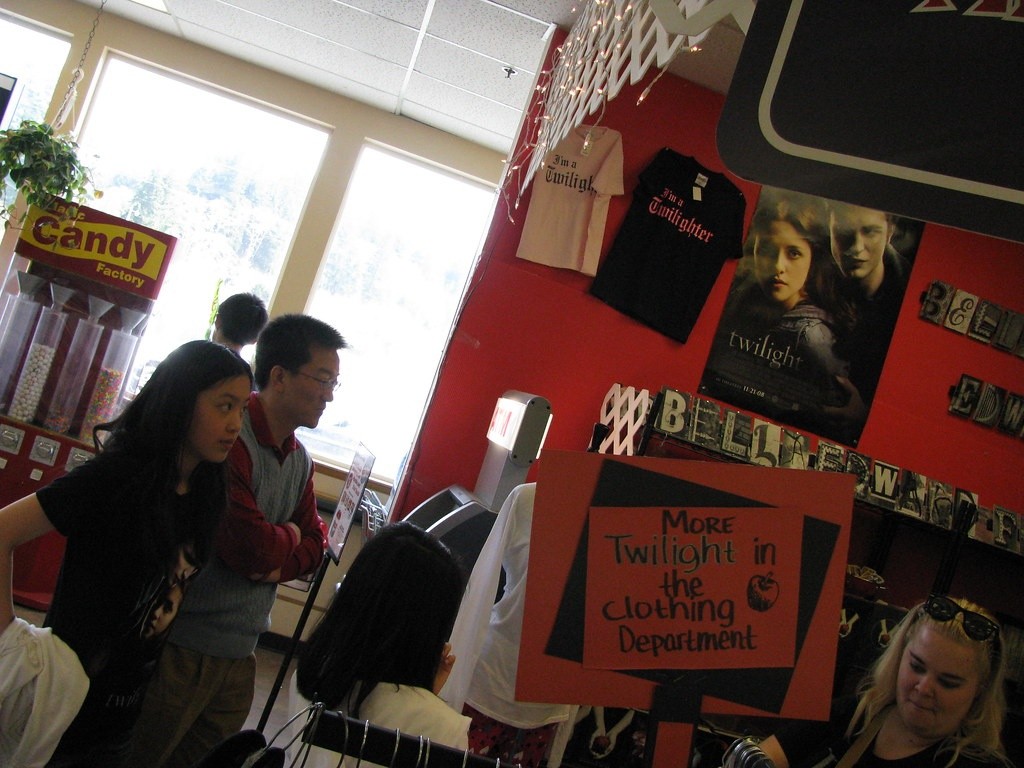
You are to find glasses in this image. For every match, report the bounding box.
[282,364,341,391]
[925,591,1001,651]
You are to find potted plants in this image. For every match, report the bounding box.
[0,119,103,250]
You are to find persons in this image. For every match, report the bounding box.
[701,204,905,436]
[288,523,472,768]
[762,600,1013,768]
[0,341,252,768]
[124,313,346,768]
[214,294,269,388]
